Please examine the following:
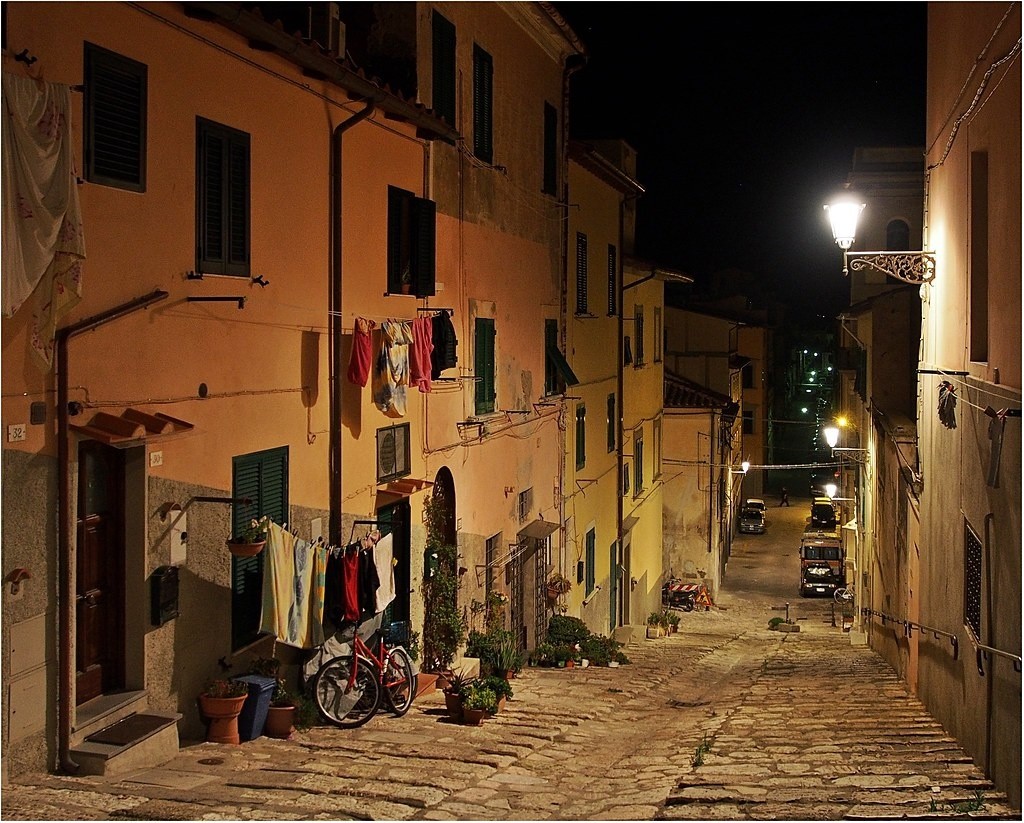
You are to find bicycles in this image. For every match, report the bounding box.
[834,582,856,604]
[313,610,418,727]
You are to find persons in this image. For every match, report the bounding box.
[778,486,790,507]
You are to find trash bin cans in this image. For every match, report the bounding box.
[235,675,277,743]
[842,615,854,632]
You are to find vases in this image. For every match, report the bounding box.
[262,704,296,740]
[199,693,246,745]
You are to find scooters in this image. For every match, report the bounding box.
[662,568,694,612]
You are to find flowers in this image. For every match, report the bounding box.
[250,659,319,730]
[205,678,247,698]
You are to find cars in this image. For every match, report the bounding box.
[738,508,765,533]
[802,563,836,598]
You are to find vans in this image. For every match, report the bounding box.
[799,529,844,581]
[811,498,837,528]
[743,498,768,511]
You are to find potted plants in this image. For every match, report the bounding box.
[462,687,496,725]
[438,667,473,720]
[528,635,630,668]
[507,639,525,679]
[430,616,466,690]
[474,677,513,714]
[547,575,569,599]
[648,610,680,638]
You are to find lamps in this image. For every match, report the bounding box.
[822,182,936,285]
